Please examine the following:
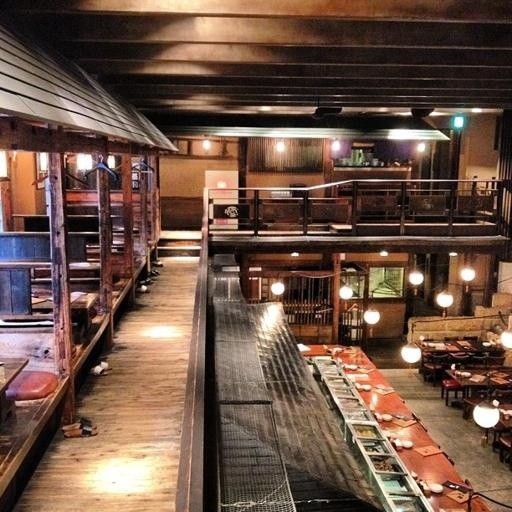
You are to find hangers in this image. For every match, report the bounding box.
[30,154,155,190]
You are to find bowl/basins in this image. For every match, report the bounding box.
[402,441,413,448]
[363,384,372,391]
[349,364,357,370]
[483,342,492,347]
[462,371,471,377]
[382,414,392,421]
[430,483,444,494]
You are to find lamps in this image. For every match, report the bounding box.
[408,254,424,285]
[502,317,512,348]
[459,252,477,281]
[340,275,352,301]
[400,322,421,363]
[473,400,500,428]
[271,274,286,296]
[434,255,454,308]
[362,292,381,325]
